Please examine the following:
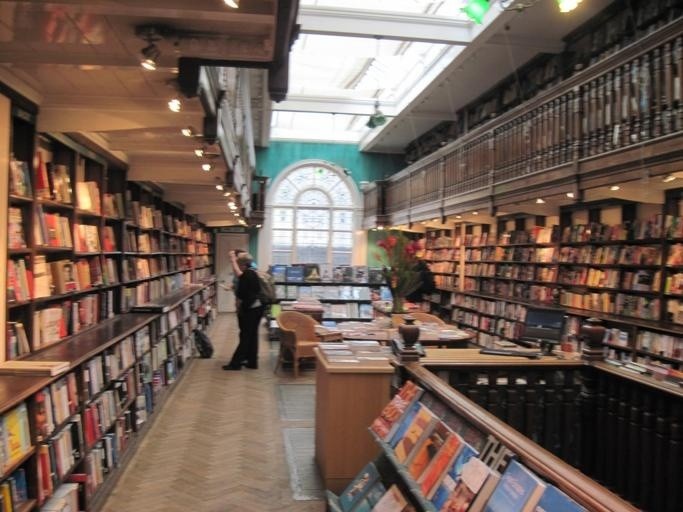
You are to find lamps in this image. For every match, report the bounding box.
[460,0,583,25]
[141,24,253,228]
[365,102,387,128]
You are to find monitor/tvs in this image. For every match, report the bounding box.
[522,305,565,356]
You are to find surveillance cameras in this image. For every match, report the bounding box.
[366,111,387,129]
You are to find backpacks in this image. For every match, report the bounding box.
[252,271,277,304]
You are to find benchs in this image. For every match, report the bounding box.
[275,309,322,379]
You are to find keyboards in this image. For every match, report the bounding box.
[479,345,537,358]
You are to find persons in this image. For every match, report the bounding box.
[221,253,266,370]
[216,248,262,361]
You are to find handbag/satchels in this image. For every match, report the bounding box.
[191,328,213,359]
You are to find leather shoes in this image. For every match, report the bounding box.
[242,361,259,369]
[223,363,242,370]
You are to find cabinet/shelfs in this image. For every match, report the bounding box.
[0,112,219,512]
[324,381,644,512]
[412,228,462,290]
[462,226,561,303]
[451,292,562,343]
[560,225,669,322]
[271,264,396,321]
[419,289,450,323]
[564,310,682,384]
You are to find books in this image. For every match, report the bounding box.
[0,144,216,512]
[399,212,682,389]
[266,263,398,366]
[333,377,589,510]
